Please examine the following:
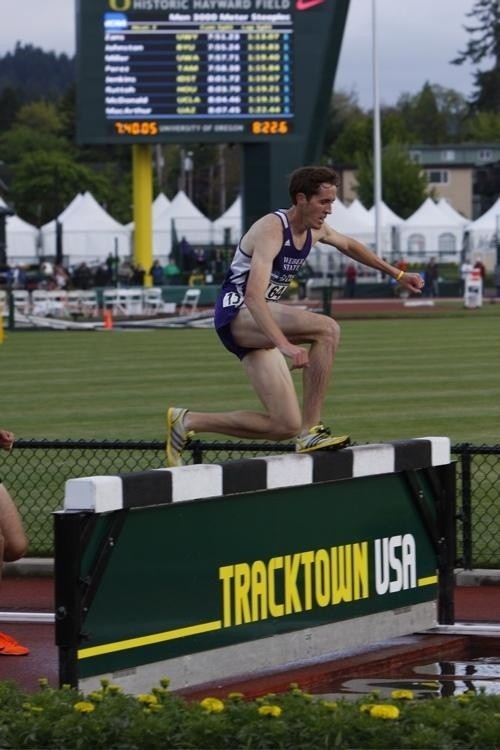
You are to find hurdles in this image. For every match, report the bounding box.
[51,437,455,695]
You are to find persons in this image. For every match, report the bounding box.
[0,234,229,286]
[331,253,499,308]
[0,427,31,656]
[163,167,428,468]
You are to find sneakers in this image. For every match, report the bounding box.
[0,630,30,656]
[165,405,196,467]
[295,421,351,455]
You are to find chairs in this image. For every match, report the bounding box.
[0,285,204,326]
